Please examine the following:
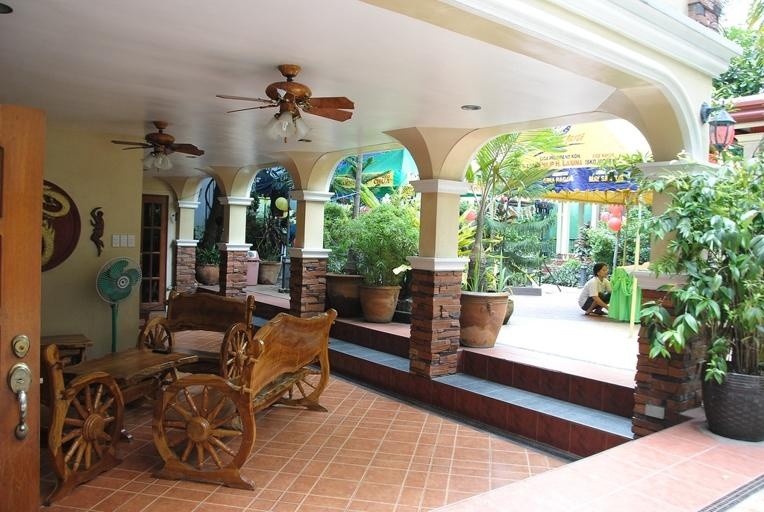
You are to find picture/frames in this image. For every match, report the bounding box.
[609,267,641,324]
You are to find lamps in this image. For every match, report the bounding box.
[142,151,173,170]
[701,99,736,154]
[265,114,310,143]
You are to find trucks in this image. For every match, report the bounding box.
[465,209,476,224]
[275,197,288,211]
[600,205,623,230]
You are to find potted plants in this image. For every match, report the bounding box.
[195,244,220,285]
[323,129,421,322]
[637,149,764,442]
[458,130,568,346]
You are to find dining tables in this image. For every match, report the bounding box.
[195,244,220,285]
[637,149,764,442]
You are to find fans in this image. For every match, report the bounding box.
[216,64,355,121]
[95,256,142,352]
[111,121,205,156]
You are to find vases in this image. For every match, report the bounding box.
[258,261,281,285]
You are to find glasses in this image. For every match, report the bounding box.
[701,99,736,154]
[142,151,173,170]
[265,114,310,143]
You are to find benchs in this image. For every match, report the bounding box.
[41,344,124,507]
[137,290,256,386]
[150,309,337,491]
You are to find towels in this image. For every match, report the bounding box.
[279,256,290,293]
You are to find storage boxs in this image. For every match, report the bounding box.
[137,290,256,386]
[150,309,337,491]
[41,344,124,507]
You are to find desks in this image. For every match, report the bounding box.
[609,267,641,324]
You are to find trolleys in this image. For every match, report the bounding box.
[95,256,142,352]
[111,121,205,156]
[216,64,355,121]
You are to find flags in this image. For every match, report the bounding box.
[258,261,281,285]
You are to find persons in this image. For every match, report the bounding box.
[90,207,104,256]
[579,263,612,317]
